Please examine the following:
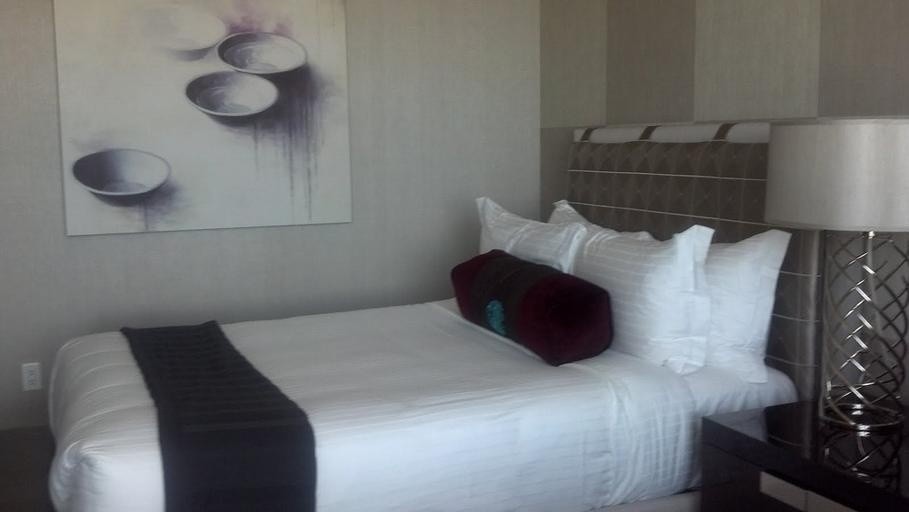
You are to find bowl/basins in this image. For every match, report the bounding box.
[209,32,308,79]
[70,148,170,206]
[183,72,279,126]
[169,13,228,60]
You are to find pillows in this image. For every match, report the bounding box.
[474,196,587,276]
[450,248,613,367]
[703,229,793,384]
[548,199,716,375]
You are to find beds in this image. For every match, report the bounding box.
[47,121,823,510]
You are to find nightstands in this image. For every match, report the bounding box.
[701,393,909,512]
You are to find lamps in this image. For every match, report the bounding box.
[763,115,908,437]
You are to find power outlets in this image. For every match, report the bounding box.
[21,362,43,392]
